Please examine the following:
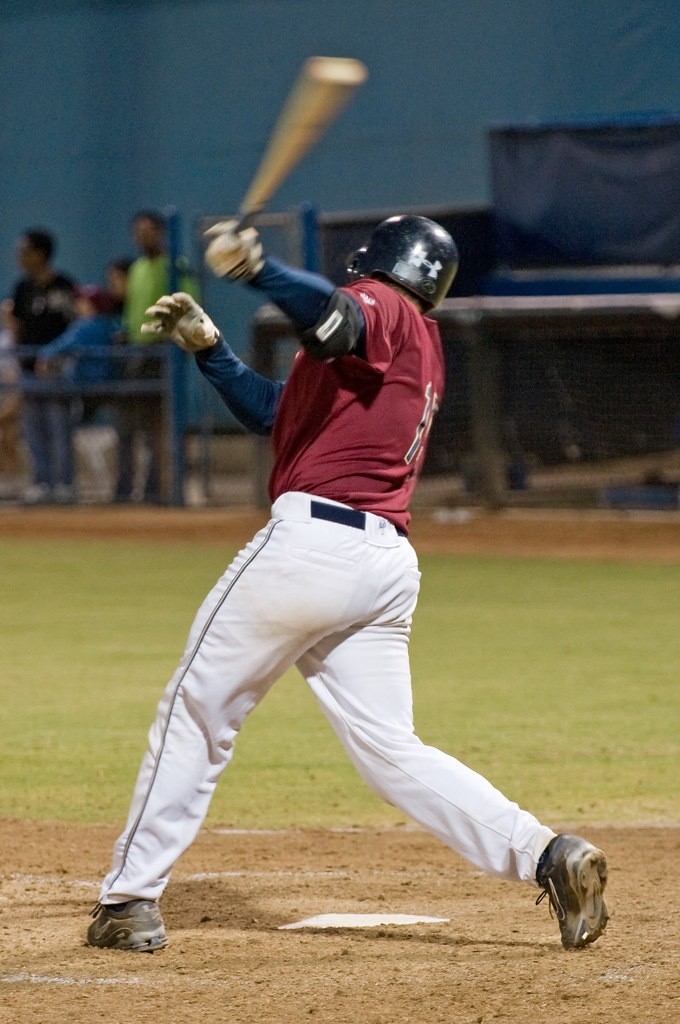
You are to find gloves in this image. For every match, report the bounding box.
[204,219,266,282]
[141,292,223,352]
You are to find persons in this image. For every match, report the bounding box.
[1,209,200,506]
[86,214,608,951]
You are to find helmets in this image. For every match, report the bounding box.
[345,215,460,308]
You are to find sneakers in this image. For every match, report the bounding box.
[112,493,166,506]
[535,834,611,951]
[21,484,74,504]
[87,900,169,952]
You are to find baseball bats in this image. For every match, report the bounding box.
[233,52,369,234]
